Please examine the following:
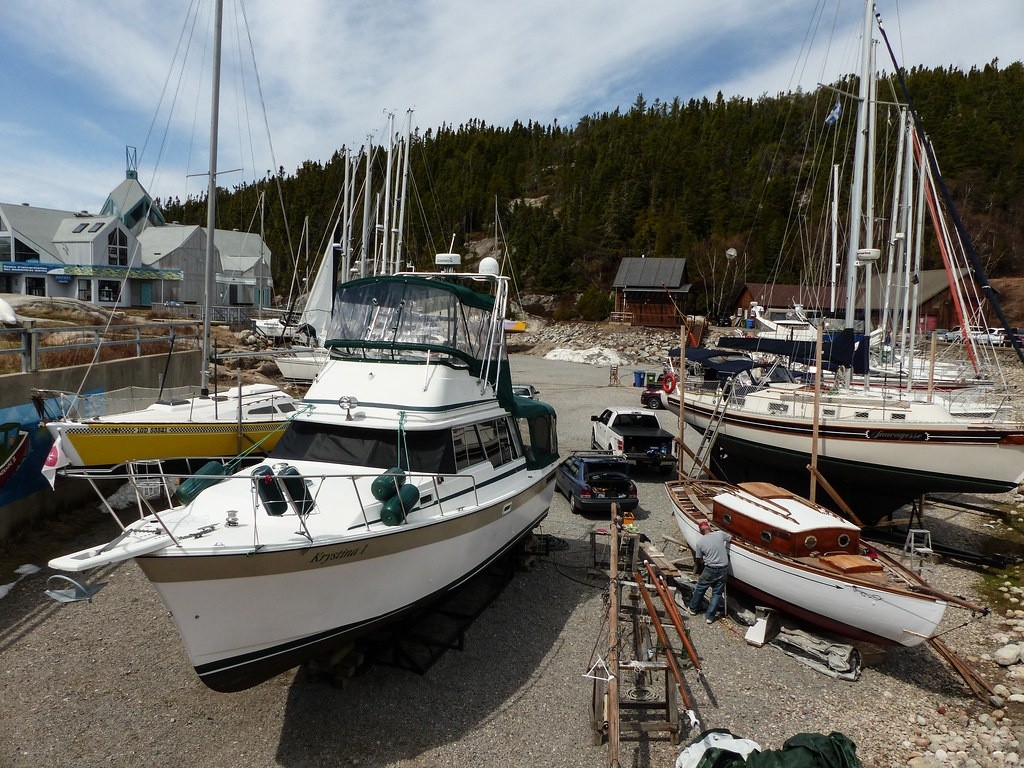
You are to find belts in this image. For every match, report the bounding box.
[707,565,729,569]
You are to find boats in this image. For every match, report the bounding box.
[52,235,560,695]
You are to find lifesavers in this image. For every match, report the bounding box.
[663,373,675,393]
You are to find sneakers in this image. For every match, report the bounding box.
[706,618,712,624]
[687,607,697,616]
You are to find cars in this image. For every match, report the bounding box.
[1001,327,1024,348]
[932,329,949,342]
[975,328,1007,346]
[922,331,932,340]
[640,388,666,410]
[945,326,983,343]
[554,449,639,515]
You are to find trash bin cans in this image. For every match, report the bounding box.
[633,370,646,387]
[746,319,754,329]
[741,319,746,328]
[647,372,656,384]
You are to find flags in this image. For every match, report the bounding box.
[41,436,70,491]
[824,100,842,125]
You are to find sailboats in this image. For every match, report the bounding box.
[652,0,1024,534]
[223,107,529,385]
[665,317,992,652]
[32,0,304,479]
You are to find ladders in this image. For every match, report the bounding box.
[898,528,935,587]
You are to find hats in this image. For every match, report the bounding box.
[699,522,708,527]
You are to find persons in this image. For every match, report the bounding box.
[688,522,733,623]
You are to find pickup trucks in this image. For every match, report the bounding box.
[590,406,679,474]
